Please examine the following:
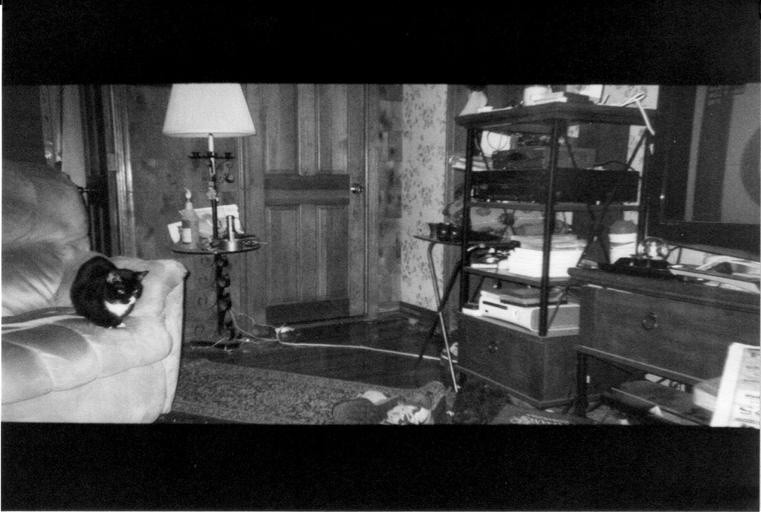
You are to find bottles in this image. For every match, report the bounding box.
[222,214,241,252]
[180,189,199,249]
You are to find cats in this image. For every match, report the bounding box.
[68,254,151,332]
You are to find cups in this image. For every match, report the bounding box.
[428,221,463,242]
[523,85,548,106]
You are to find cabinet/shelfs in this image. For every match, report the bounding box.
[456,100,660,412]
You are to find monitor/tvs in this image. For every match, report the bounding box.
[638,81,761,292]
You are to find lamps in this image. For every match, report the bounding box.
[161,83,255,248]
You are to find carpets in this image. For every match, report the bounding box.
[172,357,591,424]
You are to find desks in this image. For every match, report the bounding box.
[170,240,259,350]
[412,227,521,392]
[568,264,760,417]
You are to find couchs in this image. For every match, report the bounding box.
[2,157,189,423]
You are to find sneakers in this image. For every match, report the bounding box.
[379,376,453,425]
[440,341,459,364]
[329,384,406,424]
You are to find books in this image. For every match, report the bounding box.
[500,289,568,307]
[709,342,760,429]
[505,231,637,278]
[451,157,492,172]
[533,91,590,104]
[193,203,245,234]
